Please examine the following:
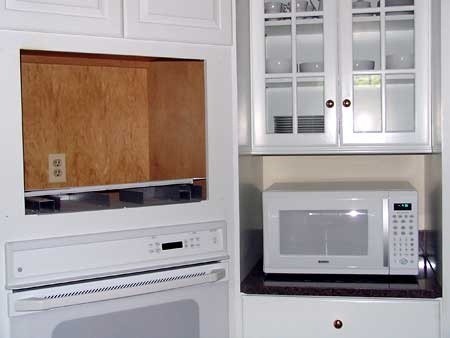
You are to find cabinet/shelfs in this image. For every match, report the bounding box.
[250,0,429,147]
[242,295,440,337]
[0,1,232,46]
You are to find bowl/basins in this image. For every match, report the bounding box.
[301,61,323,75]
[352,59,375,75]
[266,60,293,76]
[386,51,420,76]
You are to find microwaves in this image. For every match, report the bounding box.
[262,182,421,280]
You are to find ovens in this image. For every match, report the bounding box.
[1,263,230,338]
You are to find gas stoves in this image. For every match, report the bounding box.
[5,182,230,284]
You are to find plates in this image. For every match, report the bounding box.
[274,115,328,139]
